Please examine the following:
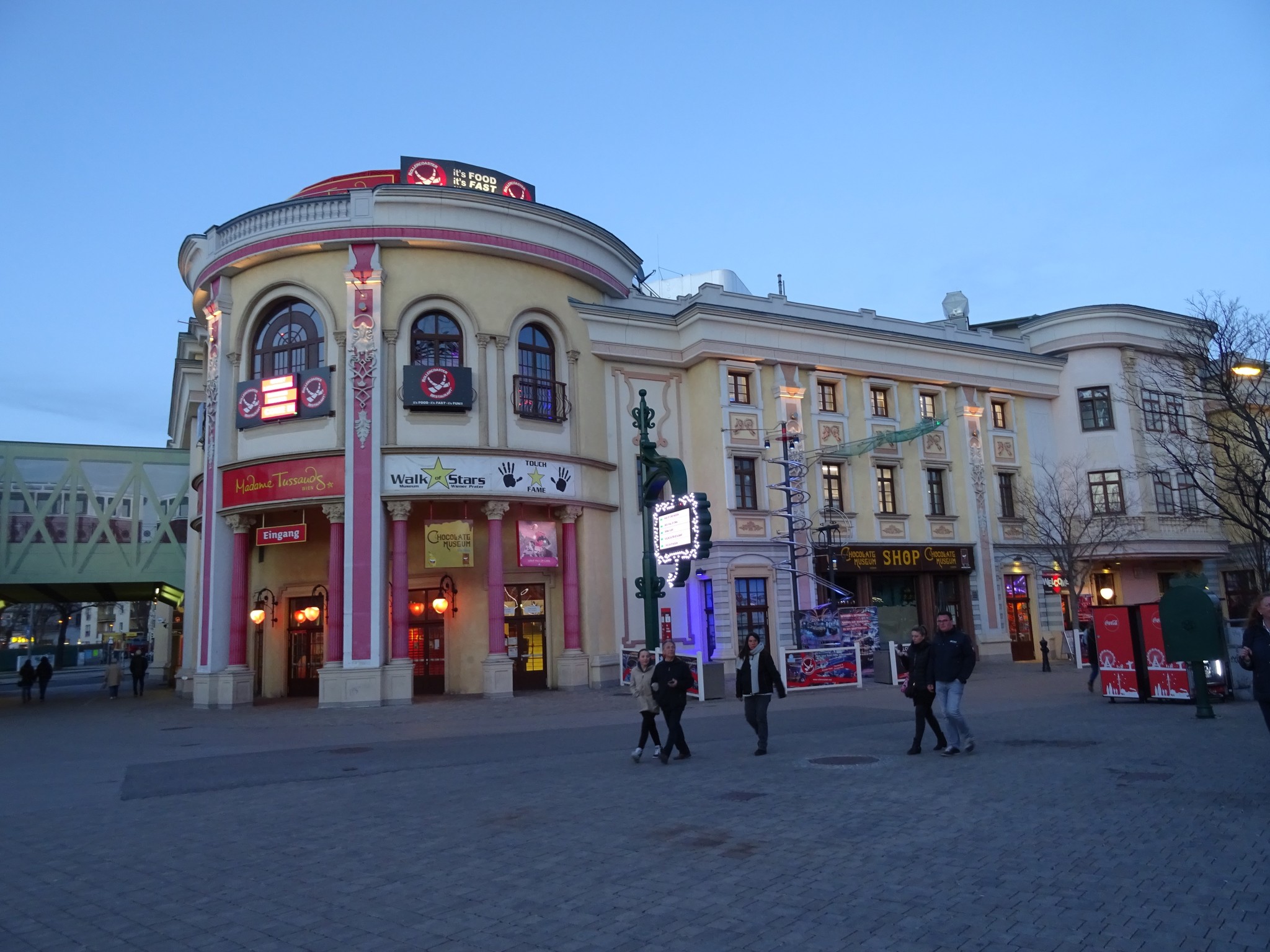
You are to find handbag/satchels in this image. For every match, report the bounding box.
[1081,629,1087,645]
[900,679,917,699]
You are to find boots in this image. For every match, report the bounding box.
[907,738,921,755]
[653,745,662,758]
[632,747,642,762]
[933,732,948,750]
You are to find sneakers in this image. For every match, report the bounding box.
[940,745,961,756]
[965,741,975,751]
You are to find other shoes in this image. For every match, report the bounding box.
[754,749,767,756]
[1087,683,1094,693]
[674,753,691,759]
[658,752,668,764]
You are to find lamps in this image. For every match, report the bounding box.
[305,585,329,625]
[433,575,457,618]
[829,553,838,562]
[696,568,706,576]
[250,588,278,628]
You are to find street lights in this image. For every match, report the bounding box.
[762,422,856,651]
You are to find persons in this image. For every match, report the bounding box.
[630,648,663,762]
[735,632,786,756]
[1086,622,1099,693]
[1238,592,1270,732]
[19,659,35,701]
[129,650,148,696]
[35,656,53,699]
[104,658,123,699]
[926,611,976,757]
[896,625,947,755]
[650,639,694,765]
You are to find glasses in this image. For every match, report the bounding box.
[937,619,950,624]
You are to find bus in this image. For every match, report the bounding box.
[102,632,149,659]
